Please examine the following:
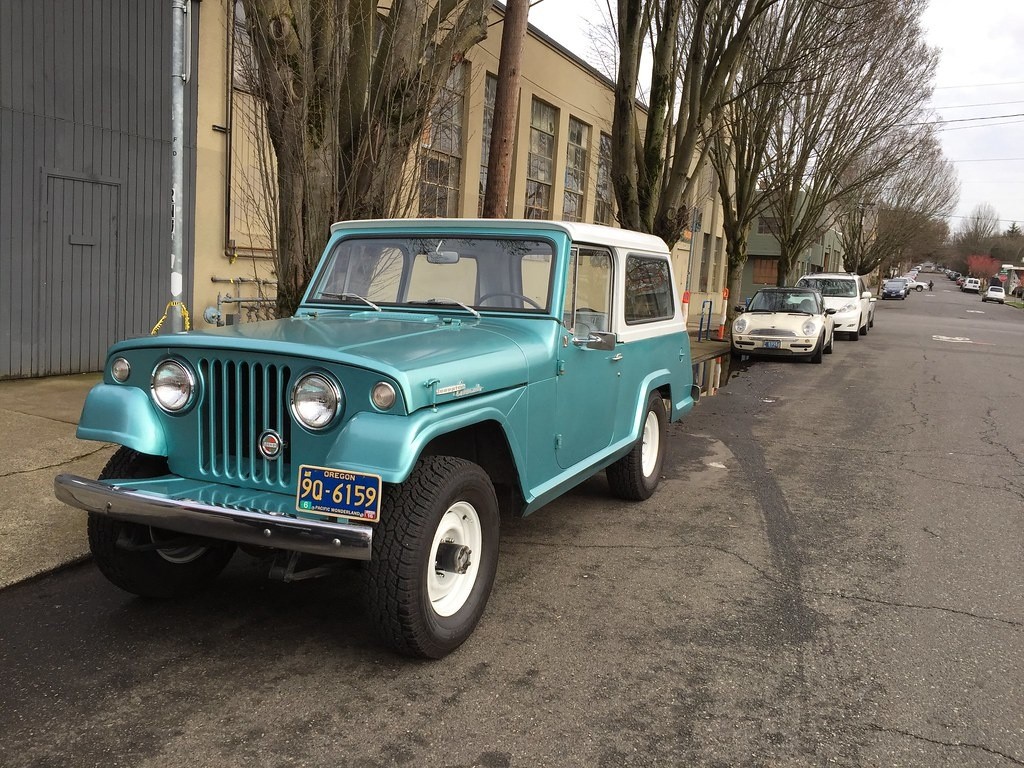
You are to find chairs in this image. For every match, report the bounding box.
[768,299,787,311]
[797,299,812,312]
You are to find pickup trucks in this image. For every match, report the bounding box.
[882,278,928,292]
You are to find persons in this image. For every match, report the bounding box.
[929,280,932,291]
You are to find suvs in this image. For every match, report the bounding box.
[981,286,1006,304]
[961,278,981,293]
[54,220,703,658]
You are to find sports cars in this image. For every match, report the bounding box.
[867,290,877,328]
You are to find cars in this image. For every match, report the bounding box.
[788,271,872,341]
[901,265,922,280]
[732,287,836,364]
[937,267,966,289]
[882,279,910,299]
